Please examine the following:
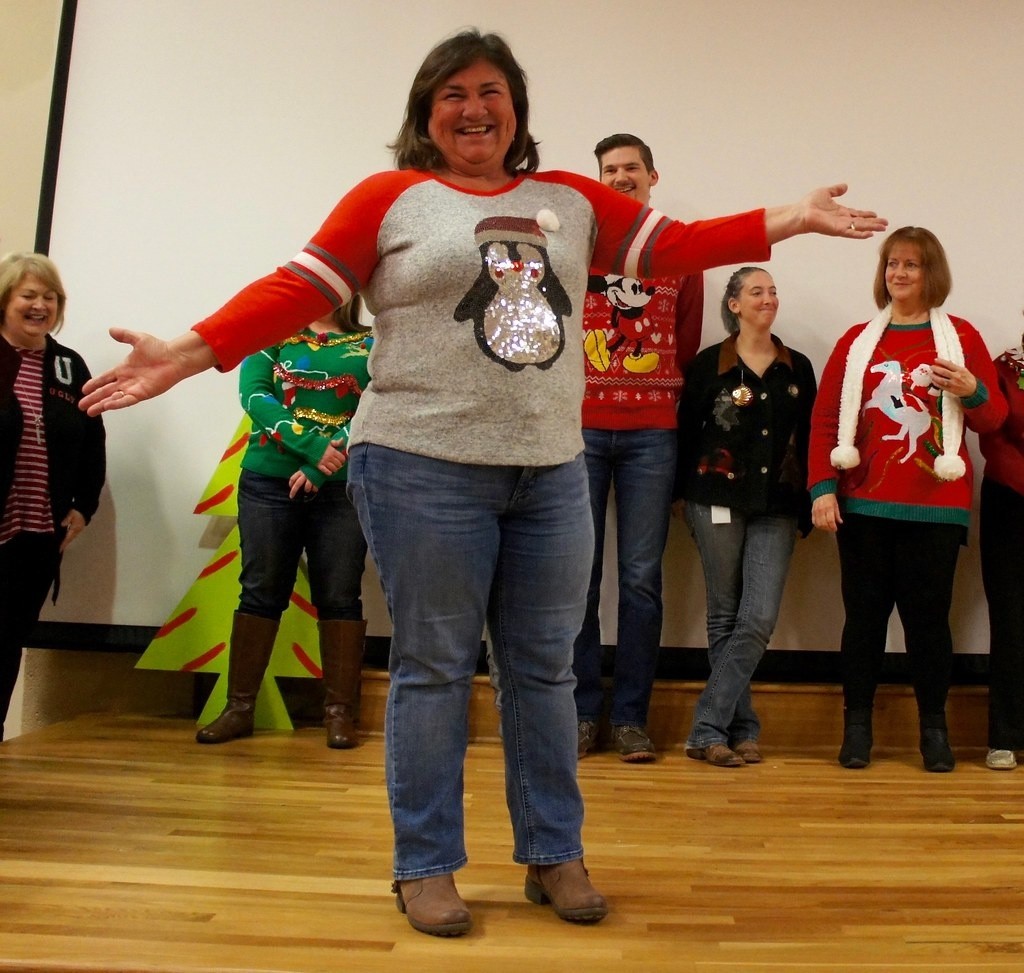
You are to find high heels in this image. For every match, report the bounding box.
[523,850,607,922]
[392,874,473,935]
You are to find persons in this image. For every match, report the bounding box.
[979,330,1024,768]
[77,28,886,936]
[575,134,705,763]
[197,295,375,749]
[807,225,1010,774]
[0,252,107,743]
[670,267,820,766]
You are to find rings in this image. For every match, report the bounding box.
[850,223,856,231]
[943,379,951,388]
[117,390,127,397]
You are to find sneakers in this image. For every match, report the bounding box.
[576,721,598,758]
[984,748,1017,769]
[918,714,956,771]
[611,722,655,762]
[838,710,873,767]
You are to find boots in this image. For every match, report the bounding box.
[318,617,367,746]
[195,611,279,744]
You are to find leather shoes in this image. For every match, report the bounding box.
[688,739,762,766]
